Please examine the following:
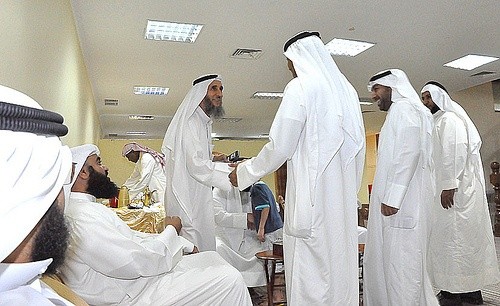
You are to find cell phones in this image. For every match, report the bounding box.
[227,150,240,161]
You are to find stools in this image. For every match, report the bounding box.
[255,250,287,306]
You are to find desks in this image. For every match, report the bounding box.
[96,205,166,234]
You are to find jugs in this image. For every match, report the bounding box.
[141,186,157,208]
[114,186,131,208]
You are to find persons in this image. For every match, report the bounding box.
[161,75,283,287]
[364,69,440,306]
[122,142,166,203]
[420,81,500,306]
[0,85,78,306]
[229,31,366,306]
[55,144,253,306]
